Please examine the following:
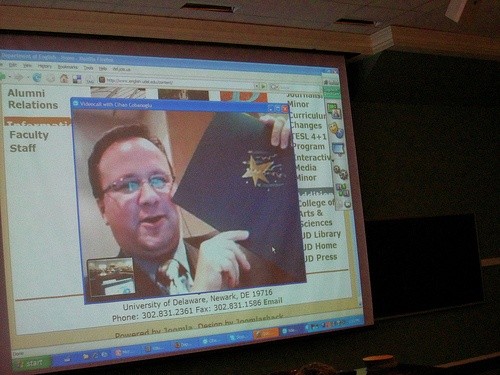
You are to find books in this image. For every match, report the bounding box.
[172,112,305,284]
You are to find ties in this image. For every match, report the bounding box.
[159,258,186,296]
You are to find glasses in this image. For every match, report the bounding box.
[99,173,175,194]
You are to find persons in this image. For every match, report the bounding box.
[85,115,303,299]
